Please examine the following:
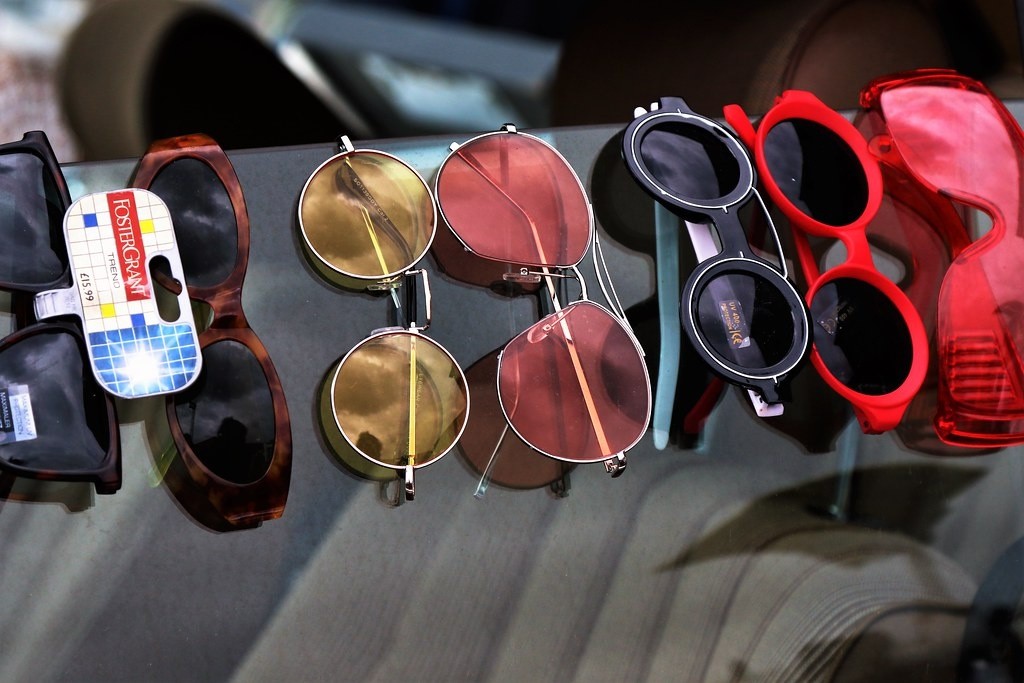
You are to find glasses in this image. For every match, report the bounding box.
[723,90,930,437]
[430,122,654,500]
[419,180,593,499]
[296,133,471,503]
[0,130,123,504]
[619,96,815,450]
[846,67,1023,449]
[129,136,292,531]
